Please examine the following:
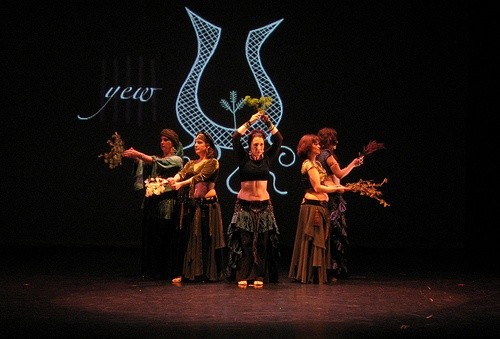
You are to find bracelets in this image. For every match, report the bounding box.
[244,120,251,129]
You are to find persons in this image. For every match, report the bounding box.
[226,111,282,285]
[164,131,226,283]
[289,134,350,284]
[122,128,184,288]
[317,127,365,276]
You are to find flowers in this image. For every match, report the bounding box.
[143,176,176,196]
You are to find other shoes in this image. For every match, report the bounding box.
[254,281,263,285]
[238,281,247,284]
[332,278,337,282]
[172,276,182,283]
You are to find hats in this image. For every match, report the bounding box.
[161,129,179,143]
